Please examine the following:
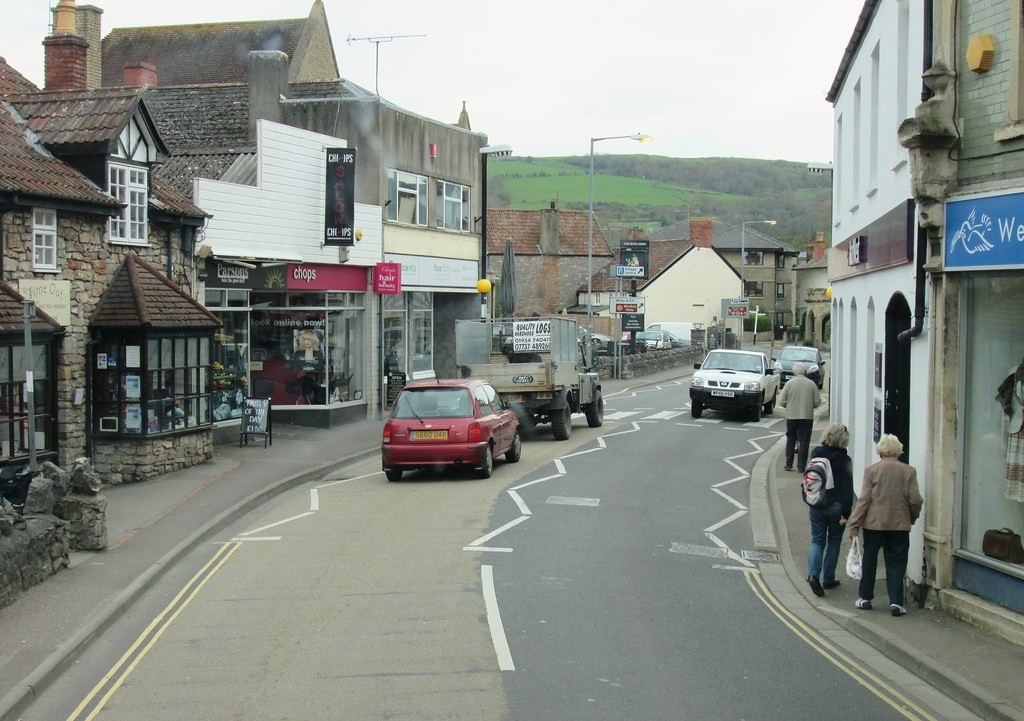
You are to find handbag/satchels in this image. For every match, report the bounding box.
[845,536,862,580]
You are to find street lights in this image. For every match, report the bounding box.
[738,220,778,349]
[583,134,654,369]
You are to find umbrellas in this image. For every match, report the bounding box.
[501,238,519,336]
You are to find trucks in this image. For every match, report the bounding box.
[455,315,604,440]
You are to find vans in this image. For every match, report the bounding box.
[647,322,703,346]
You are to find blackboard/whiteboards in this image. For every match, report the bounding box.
[240,396,271,435]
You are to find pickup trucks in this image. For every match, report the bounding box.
[691,350,781,423]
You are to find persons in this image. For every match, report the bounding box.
[995,357,1024,502]
[779,363,822,473]
[807,424,853,597]
[849,434,924,617]
[292,331,324,372]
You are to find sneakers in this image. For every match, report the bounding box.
[889,604,907,616]
[855,598,871,609]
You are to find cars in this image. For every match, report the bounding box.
[382,378,520,482]
[580,326,615,355]
[772,346,826,390]
[622,331,690,350]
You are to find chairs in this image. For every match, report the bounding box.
[251,376,273,397]
[453,394,474,415]
[421,396,438,411]
[286,376,316,405]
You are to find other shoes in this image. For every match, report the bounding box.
[823,579,840,589]
[808,575,824,596]
[784,464,792,469]
[798,467,804,473]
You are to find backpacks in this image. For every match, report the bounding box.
[801,452,843,508]
